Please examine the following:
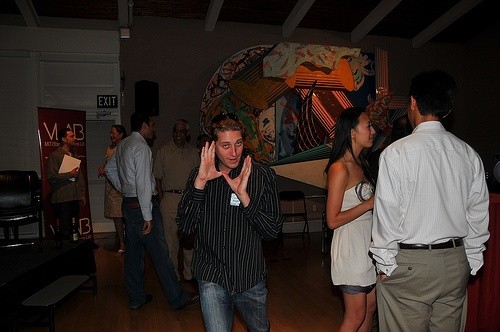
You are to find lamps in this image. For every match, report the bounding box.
[120,28,130,38]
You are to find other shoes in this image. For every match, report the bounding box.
[129,292,151,308]
[172,290,195,309]
[118,248,124,254]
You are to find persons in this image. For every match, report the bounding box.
[47,127,84,240]
[104,111,200,311]
[176,113,283,332]
[154,119,195,284]
[369,70,491,332]
[323,108,379,332]
[98,124,128,253]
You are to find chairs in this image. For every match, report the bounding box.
[279,191,310,253]
[0,170,44,252]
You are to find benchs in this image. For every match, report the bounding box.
[10,273,97,332]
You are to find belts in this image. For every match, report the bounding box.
[168,189,184,194]
[399,238,463,250]
